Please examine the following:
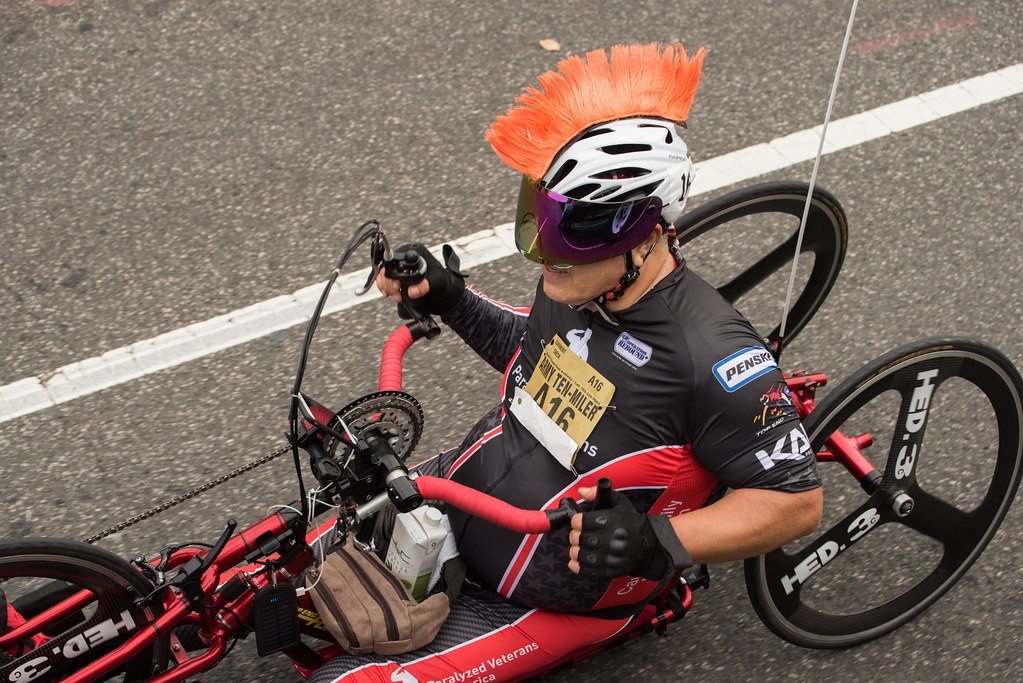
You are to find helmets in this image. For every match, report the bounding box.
[485,44,697,270]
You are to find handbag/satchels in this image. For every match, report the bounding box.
[305,527,465,656]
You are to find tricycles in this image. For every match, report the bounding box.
[0,179,1023,683]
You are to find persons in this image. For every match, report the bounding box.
[305,43,823,683]
